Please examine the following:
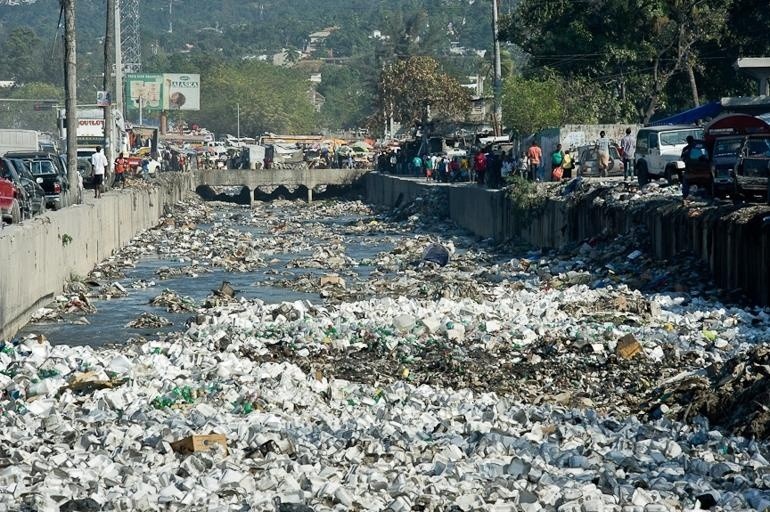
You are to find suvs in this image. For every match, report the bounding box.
[633,125,706,187]
[710,133,770,205]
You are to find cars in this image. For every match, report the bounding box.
[165,130,395,168]
[574,145,624,178]
[0,98,166,225]
[382,127,511,182]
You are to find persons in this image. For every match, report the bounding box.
[348,150,353,169]
[141,152,151,182]
[594,130,615,178]
[682,136,707,173]
[91,146,109,198]
[377,139,576,189]
[621,127,637,182]
[179,152,185,172]
[111,152,126,189]
